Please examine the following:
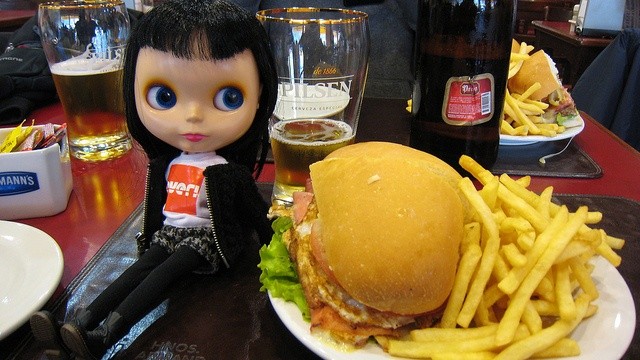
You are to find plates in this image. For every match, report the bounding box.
[422,114,585,146]
[266,253,636,359]
[0,220,65,339]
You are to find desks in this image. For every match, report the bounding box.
[530,19,613,93]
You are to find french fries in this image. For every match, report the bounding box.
[498,41,567,137]
[377,153,626,359]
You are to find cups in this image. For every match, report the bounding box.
[38,1,133,164]
[256,7,371,213]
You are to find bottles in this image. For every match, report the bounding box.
[409,1,514,180]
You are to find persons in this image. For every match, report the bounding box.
[28,2,275,360]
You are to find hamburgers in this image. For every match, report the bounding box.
[508,48,579,119]
[255,140,469,351]
